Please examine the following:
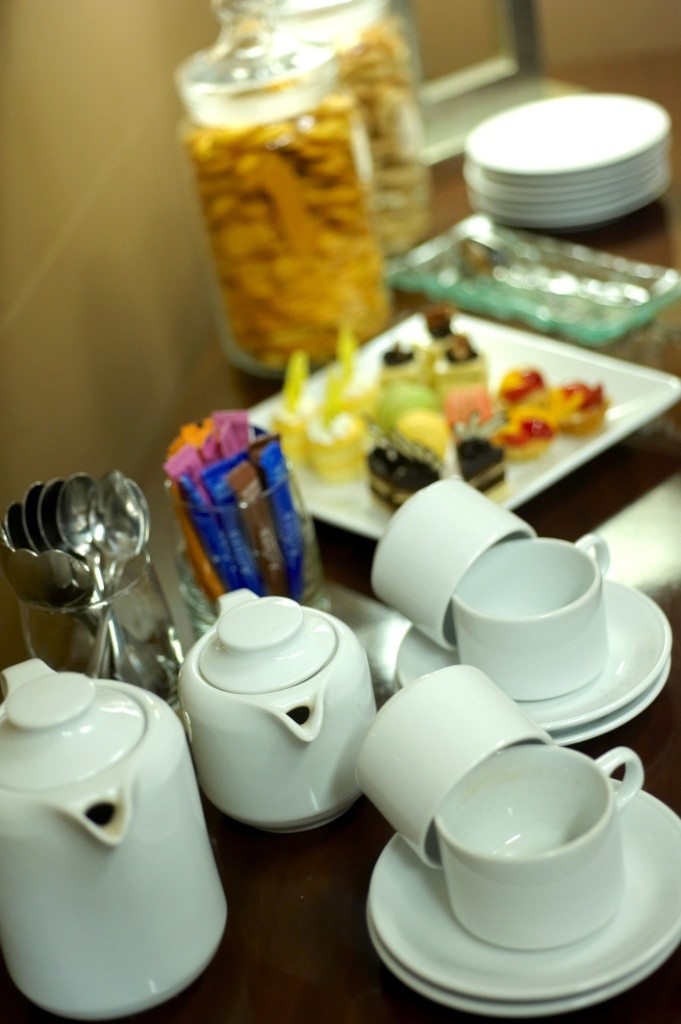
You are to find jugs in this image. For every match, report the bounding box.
[4,648,227,1020]
[181,580,377,838]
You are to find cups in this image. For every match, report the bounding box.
[159,470,336,641]
[339,481,649,954]
[2,550,179,734]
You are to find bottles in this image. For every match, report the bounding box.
[184,0,430,389]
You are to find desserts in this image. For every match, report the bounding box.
[276,306,608,507]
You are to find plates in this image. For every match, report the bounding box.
[361,783,681,1020]
[388,217,681,348]
[386,580,676,748]
[206,307,679,535]
[466,92,670,231]
